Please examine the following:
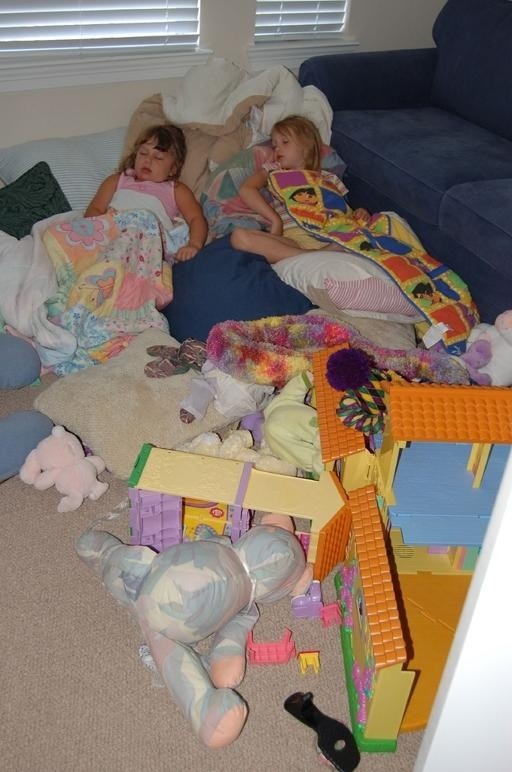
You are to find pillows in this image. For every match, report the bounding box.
[158,230,319,343]
[271,251,415,317]
[31,327,255,480]
[121,94,165,161]
[1,161,73,241]
[199,139,346,238]
[1,126,127,211]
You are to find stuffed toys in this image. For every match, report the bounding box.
[20,310,512,751]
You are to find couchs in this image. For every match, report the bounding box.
[299,0,512,326]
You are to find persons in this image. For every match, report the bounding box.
[83,124,209,262]
[229,114,372,263]
